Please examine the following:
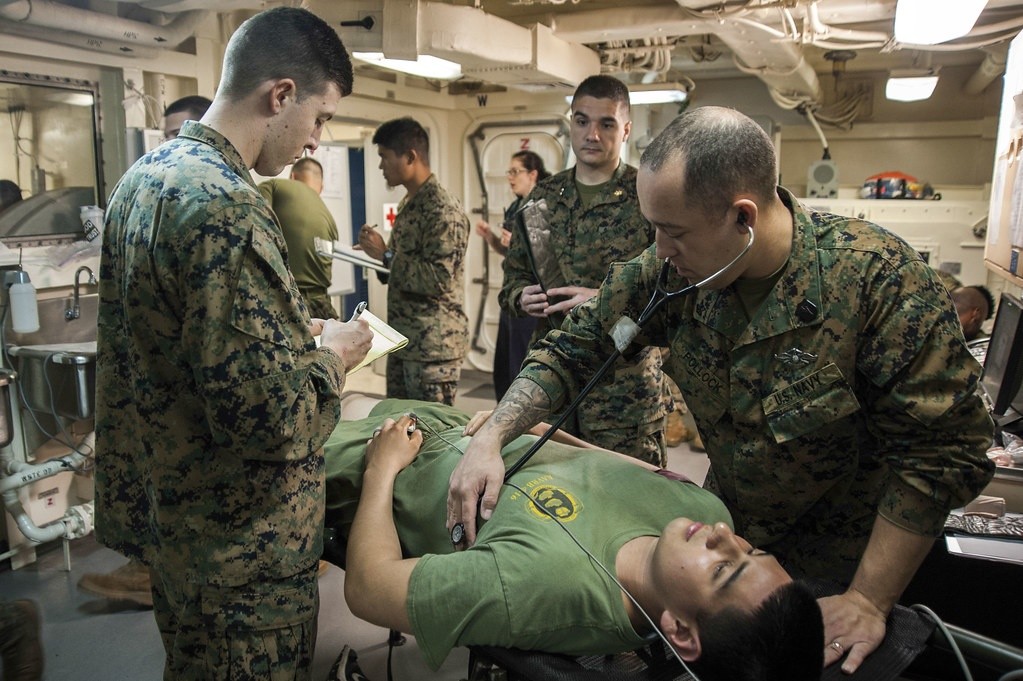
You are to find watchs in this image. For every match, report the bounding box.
[383,249,394,268]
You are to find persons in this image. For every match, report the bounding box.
[498,75,676,467]
[477,150,553,405]
[257,158,339,321]
[92,5,371,681]
[932,269,994,341]
[78,96,213,607]
[444,107,998,674]
[357,116,470,407]
[312,392,826,681]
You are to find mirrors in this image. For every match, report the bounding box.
[0,69,106,243]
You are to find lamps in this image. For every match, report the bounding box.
[886,70,939,103]
[564,83,688,109]
[894,0,989,44]
[353,50,462,81]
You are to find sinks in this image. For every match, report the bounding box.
[16,340,98,421]
[0,367,19,448]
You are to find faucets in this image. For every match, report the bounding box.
[64,265,98,321]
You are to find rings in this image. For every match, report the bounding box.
[832,642,844,650]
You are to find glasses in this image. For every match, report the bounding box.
[508,168,530,175]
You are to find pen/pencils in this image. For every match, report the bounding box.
[370,224,378,229]
[348,302,367,322]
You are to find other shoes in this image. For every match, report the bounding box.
[666,419,693,446]
[691,433,706,453]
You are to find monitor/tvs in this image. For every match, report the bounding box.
[978,290,1023,445]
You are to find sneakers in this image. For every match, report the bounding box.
[319,561,328,577]
[328,644,367,681]
[78,559,153,605]
